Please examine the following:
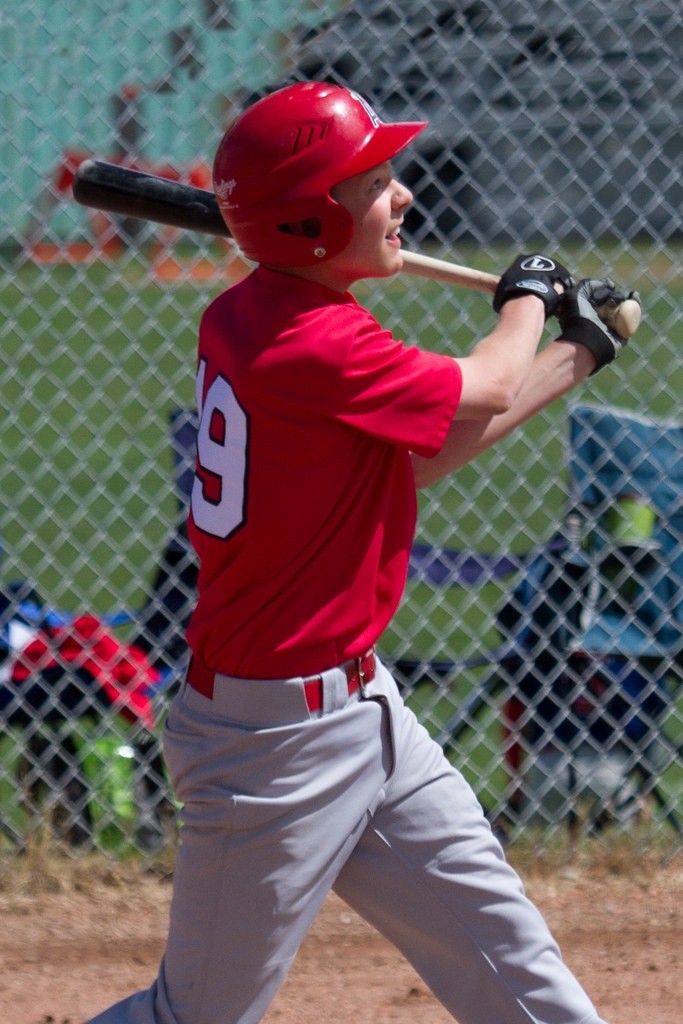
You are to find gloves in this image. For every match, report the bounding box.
[492,251,573,321]
[556,276,643,376]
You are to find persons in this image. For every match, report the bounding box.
[85,81,641,1024]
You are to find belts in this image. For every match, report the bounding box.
[188,653,379,715]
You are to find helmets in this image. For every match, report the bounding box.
[212,81,428,266]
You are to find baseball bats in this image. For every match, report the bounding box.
[70,158,644,340]
